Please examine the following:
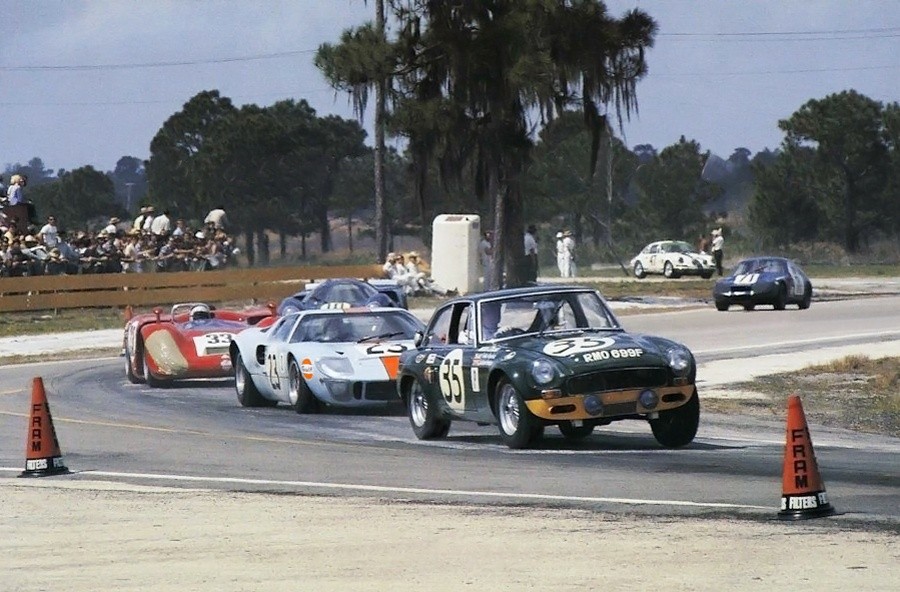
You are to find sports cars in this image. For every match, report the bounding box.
[122,302,260,387]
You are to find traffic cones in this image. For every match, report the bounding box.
[774,394,836,520]
[15,376,75,479]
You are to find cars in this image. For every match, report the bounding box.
[711,256,812,310]
[227,275,430,414]
[630,240,717,279]
[396,283,701,449]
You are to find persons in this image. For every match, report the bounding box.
[383,251,435,298]
[699,228,724,277]
[557,231,577,278]
[320,316,343,340]
[458,305,512,345]
[185,305,209,329]
[524,223,538,285]
[478,229,495,292]
[0,174,240,295]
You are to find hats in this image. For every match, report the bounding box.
[9,220,17,229]
[97,233,106,238]
[564,231,572,235]
[148,207,153,212]
[409,251,418,257]
[388,252,400,260]
[557,232,563,237]
[49,248,61,257]
[109,217,120,223]
[139,208,148,213]
[528,225,536,231]
[711,230,718,235]
[25,235,33,241]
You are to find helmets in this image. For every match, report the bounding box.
[189,305,210,321]
[195,231,206,239]
[320,318,343,339]
